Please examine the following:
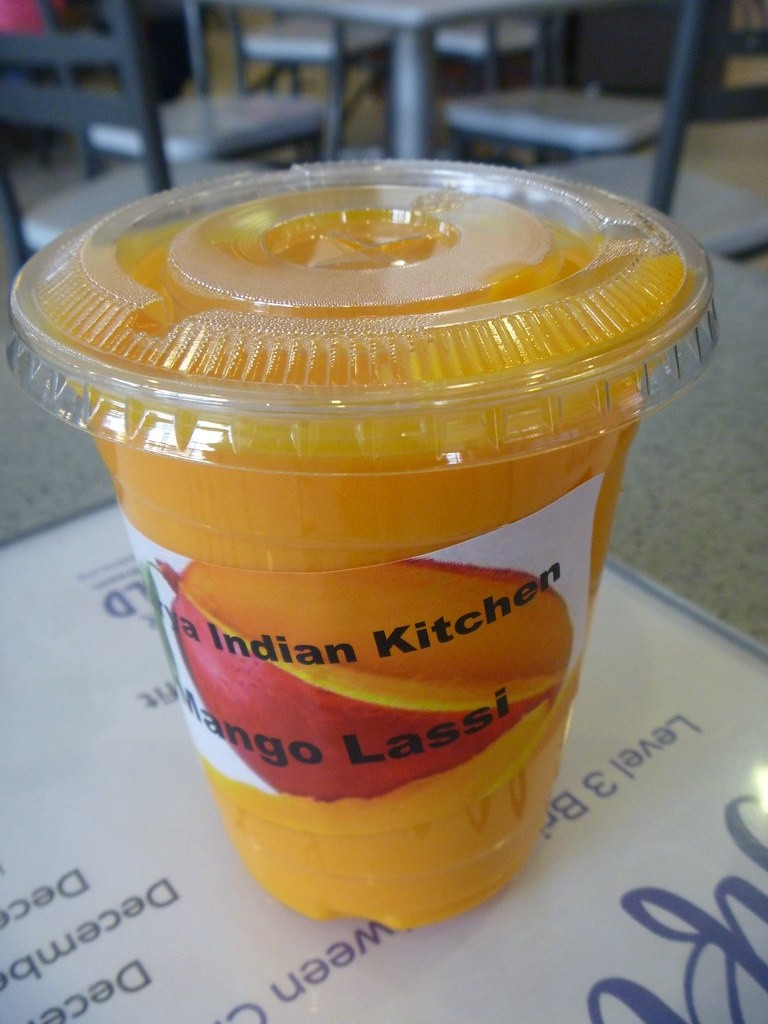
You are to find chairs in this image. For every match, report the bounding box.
[439,0,682,173]
[523,0,767,266]
[39,1,331,169]
[0,0,292,270]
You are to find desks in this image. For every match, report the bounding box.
[0,492,768,1023]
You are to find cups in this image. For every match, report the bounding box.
[9,158,713,931]
